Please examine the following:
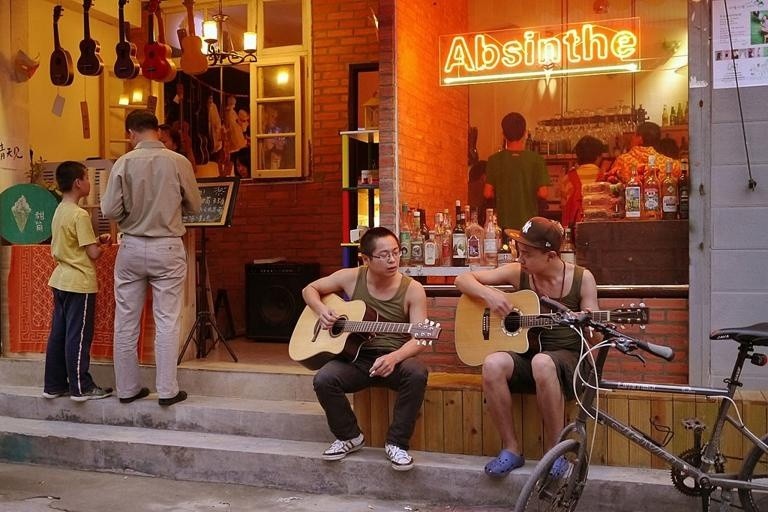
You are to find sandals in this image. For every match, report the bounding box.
[548,455,569,480]
[484,449,526,478]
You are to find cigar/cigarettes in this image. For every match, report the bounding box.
[366,368,378,377]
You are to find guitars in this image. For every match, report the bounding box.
[180,0,209,76]
[142,1,177,83]
[454,284,649,367]
[288,292,442,371]
[171,82,210,165]
[50,5,74,86]
[77,0,105,76]
[114,0,140,79]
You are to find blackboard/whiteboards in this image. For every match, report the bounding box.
[180,182,234,226]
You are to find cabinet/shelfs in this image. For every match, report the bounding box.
[536,121,689,166]
[340,128,381,278]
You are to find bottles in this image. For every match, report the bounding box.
[662,103,689,126]
[397,200,518,269]
[526,98,638,155]
[624,156,689,220]
[560,228,576,264]
[638,104,645,123]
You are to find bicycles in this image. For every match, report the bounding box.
[515,293,768,512]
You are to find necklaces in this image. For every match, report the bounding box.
[528,261,566,314]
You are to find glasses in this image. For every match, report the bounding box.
[370,250,403,260]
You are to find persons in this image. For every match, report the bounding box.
[454,217,606,477]
[101,109,200,406]
[468,159,487,221]
[655,137,680,161]
[604,123,684,221]
[485,111,553,231]
[156,124,182,155]
[560,138,610,234]
[758,15,768,40]
[42,163,113,403]
[231,147,249,178]
[301,228,431,467]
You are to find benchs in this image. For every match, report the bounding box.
[353,365,766,475]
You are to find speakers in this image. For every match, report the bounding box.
[244,262,320,342]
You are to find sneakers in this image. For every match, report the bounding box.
[321,432,367,462]
[43,383,114,402]
[158,390,187,406]
[119,388,151,404]
[382,443,417,473]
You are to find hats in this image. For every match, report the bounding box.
[503,216,562,250]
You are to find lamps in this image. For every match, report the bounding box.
[201,1,259,69]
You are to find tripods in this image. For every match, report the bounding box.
[177,227,238,365]
